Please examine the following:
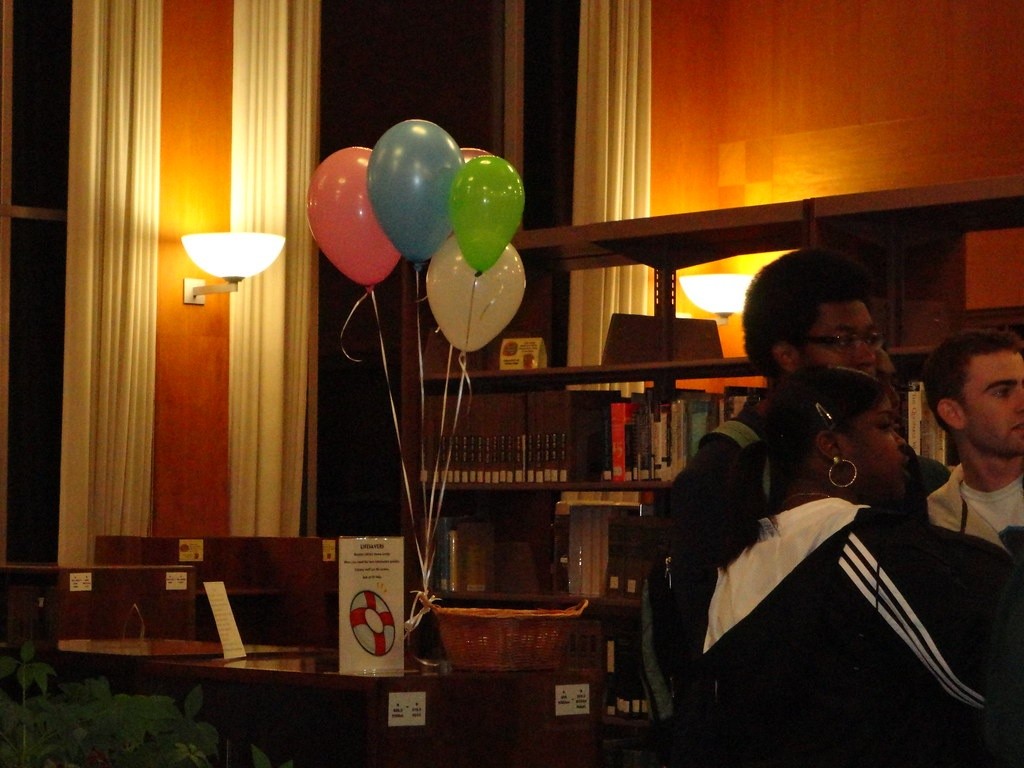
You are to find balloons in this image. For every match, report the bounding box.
[306,146,402,294]
[366,118,466,272]
[450,156,524,277]
[444,147,494,239]
[425,234,526,356]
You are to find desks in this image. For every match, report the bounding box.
[46,639,603,768]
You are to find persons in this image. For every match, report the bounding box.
[923,330,1024,562]
[659,248,885,655]
[868,347,951,524]
[699,366,1014,768]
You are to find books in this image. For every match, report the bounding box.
[597,385,770,482]
[426,491,660,599]
[902,381,950,467]
[421,395,566,484]
[573,620,651,719]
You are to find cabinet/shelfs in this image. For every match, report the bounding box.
[401,174,1022,768]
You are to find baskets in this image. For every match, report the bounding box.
[417,591,589,672]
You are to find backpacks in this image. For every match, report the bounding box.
[638,418,776,732]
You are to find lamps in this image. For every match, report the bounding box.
[179,232,286,307]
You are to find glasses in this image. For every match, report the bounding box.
[796,333,886,357]
